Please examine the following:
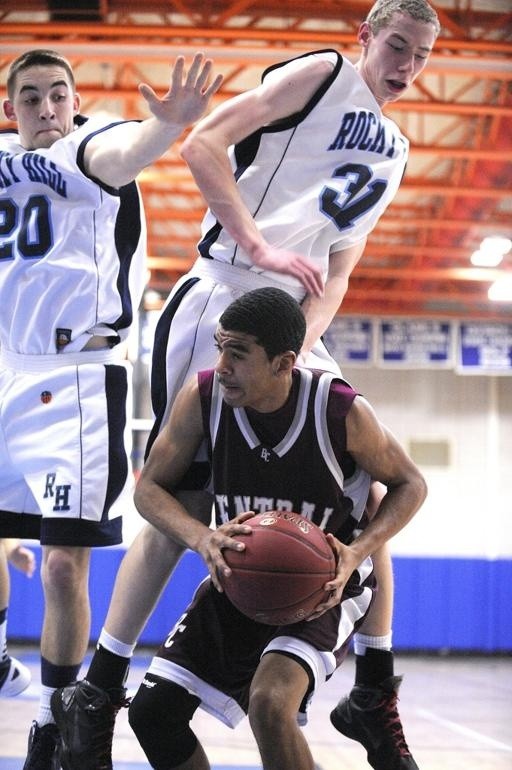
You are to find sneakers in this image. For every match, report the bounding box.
[23,720,59,770]
[330,676,419,770]
[51,679,132,769]
[0,657,32,697]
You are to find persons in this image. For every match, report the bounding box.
[122,286,429,770]
[0,47,224,763]
[45,0,452,770]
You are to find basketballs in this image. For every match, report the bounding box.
[217,512,335,624]
